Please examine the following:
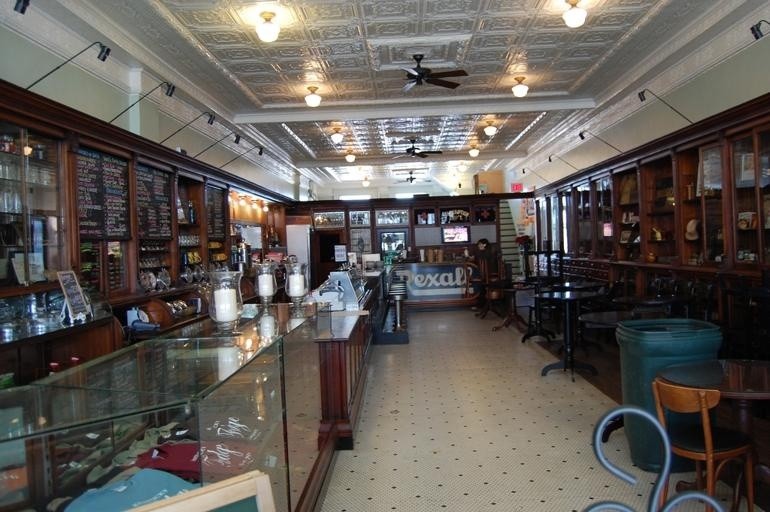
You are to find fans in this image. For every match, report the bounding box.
[393,172,431,183]
[392,140,442,160]
[399,54,469,92]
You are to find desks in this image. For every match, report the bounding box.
[659,358,770,495]
[468,275,680,383]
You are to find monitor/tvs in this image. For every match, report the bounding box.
[440,226,472,243]
[377,229,410,256]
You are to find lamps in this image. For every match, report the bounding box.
[331,128,343,143]
[484,121,498,136]
[362,177,370,187]
[562,0,587,28]
[469,144,480,156]
[27,41,109,91]
[639,89,693,125]
[750,20,770,40]
[238,3,295,42]
[511,77,530,98]
[345,150,355,162]
[14,0,30,15]
[459,162,467,172]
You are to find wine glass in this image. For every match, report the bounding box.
[253,263,278,310]
[205,271,244,337]
[283,262,312,310]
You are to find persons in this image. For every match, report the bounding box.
[469,237,498,312]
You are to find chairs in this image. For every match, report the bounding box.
[652,377,754,512]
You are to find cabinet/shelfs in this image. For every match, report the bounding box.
[0,80,331,512]
[533,93,770,421]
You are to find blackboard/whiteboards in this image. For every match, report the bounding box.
[207,187,226,238]
[77,144,172,240]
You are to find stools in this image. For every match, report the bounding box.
[388,265,408,331]
